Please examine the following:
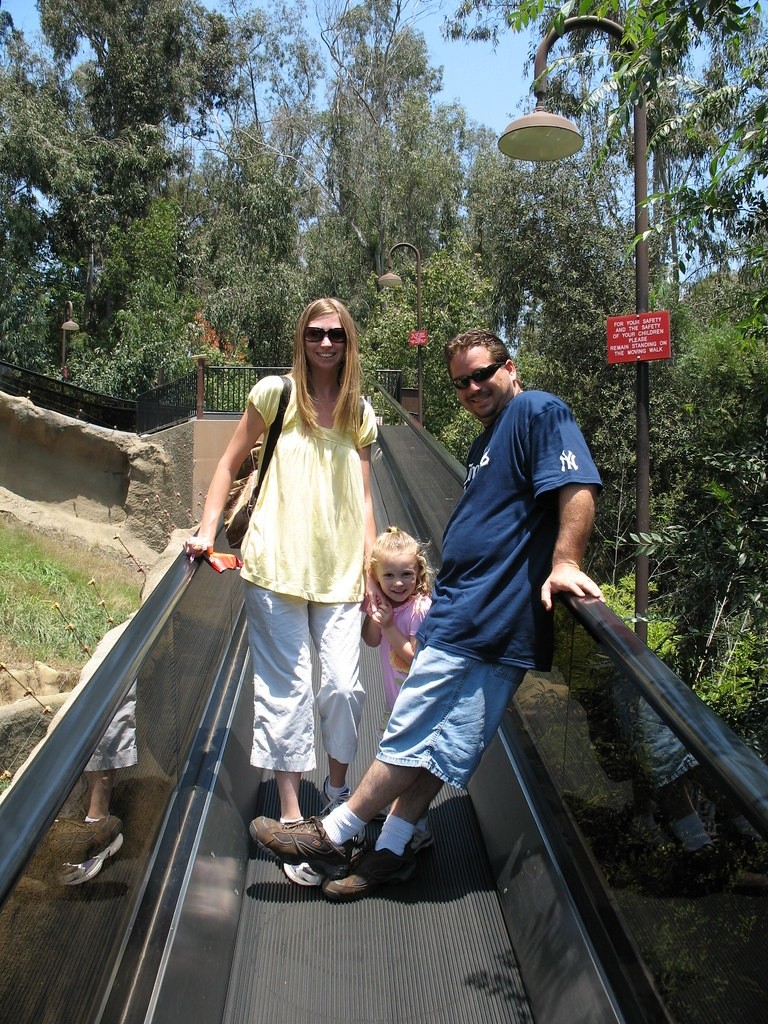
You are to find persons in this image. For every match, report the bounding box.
[185,298,378,885]
[249,330,605,899]
[58,681,137,885]
[608,668,715,852]
[361,525,434,854]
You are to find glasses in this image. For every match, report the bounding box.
[303,327,347,344]
[452,361,505,389]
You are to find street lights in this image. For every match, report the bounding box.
[378,242,423,426]
[61,300,79,382]
[497,15,649,647]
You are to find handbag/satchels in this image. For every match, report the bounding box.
[224,376,292,548]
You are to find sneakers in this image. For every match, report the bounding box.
[375,807,390,819]
[322,847,417,900]
[318,775,352,812]
[59,817,122,884]
[278,818,325,886]
[408,827,435,853]
[250,816,364,880]
